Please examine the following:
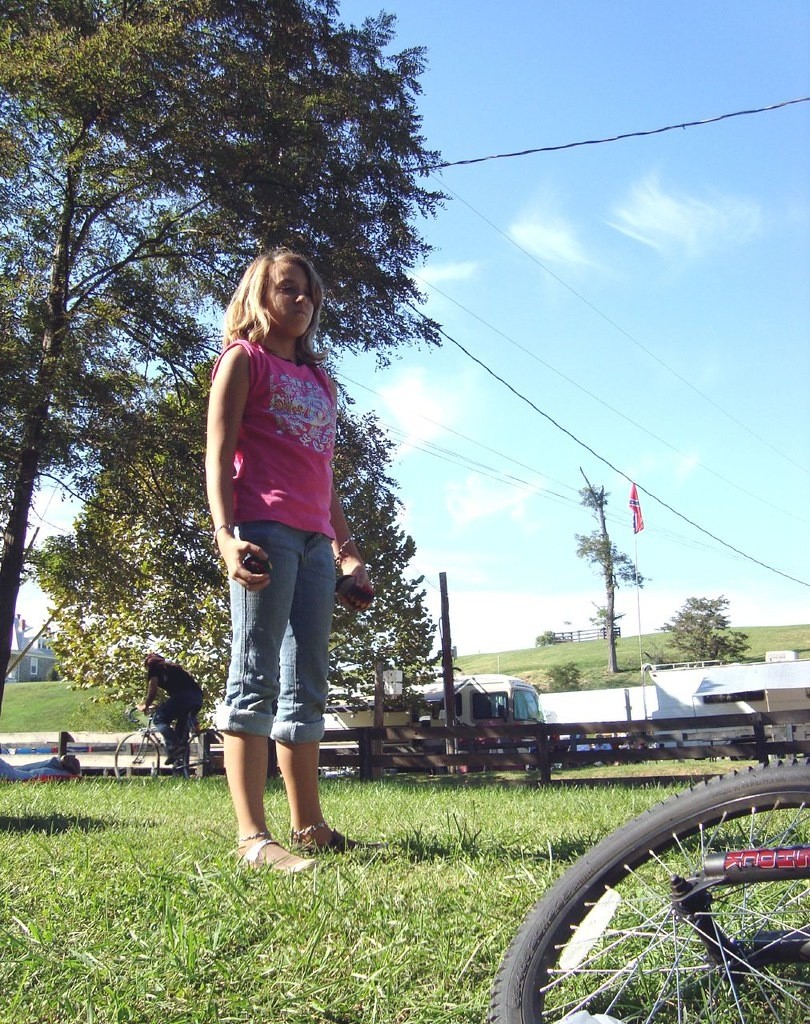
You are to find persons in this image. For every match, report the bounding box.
[135,653,204,781]
[204,246,383,871]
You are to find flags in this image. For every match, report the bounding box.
[628,483,645,535]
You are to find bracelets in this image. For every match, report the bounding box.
[211,525,236,556]
[335,537,358,571]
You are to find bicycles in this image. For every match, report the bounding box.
[113,699,226,778]
[481,756,810,1024]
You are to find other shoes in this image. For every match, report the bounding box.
[164,746,185,765]
[290,827,386,859]
[238,830,319,876]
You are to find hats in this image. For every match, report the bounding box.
[144,653,165,666]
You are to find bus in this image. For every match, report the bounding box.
[318,665,545,776]
